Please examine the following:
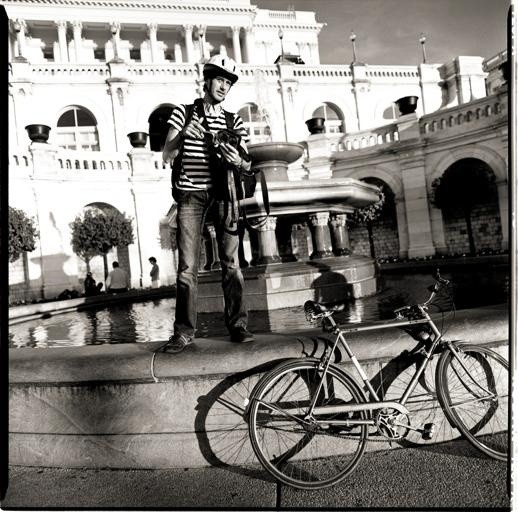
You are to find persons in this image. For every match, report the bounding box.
[161,53,254,354]
[85,261,129,296]
[148,257,159,288]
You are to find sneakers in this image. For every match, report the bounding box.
[164,332,194,353]
[229,327,256,343]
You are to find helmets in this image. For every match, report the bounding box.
[203,54,241,83]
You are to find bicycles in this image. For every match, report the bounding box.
[242,268,508,490]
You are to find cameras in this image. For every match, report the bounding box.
[217,129,241,151]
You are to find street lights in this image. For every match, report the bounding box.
[278,28,290,64]
[11,18,27,62]
[196,26,206,62]
[109,20,124,63]
[418,35,428,63]
[348,34,363,66]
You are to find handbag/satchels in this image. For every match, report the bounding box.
[212,162,270,232]
